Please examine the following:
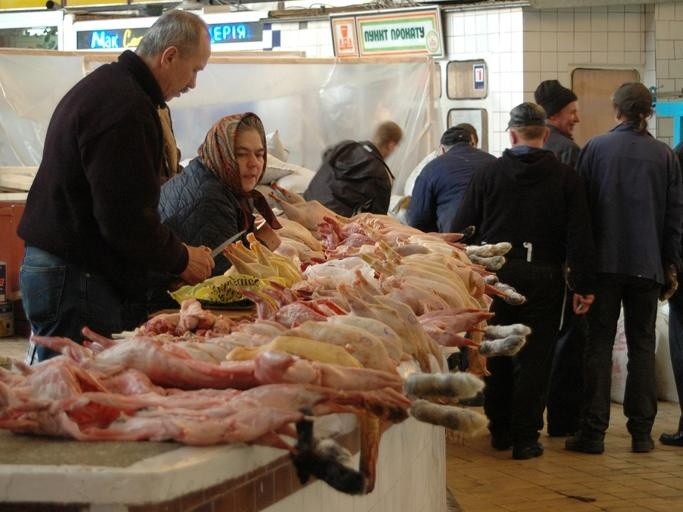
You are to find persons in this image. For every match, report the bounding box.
[18,10,216,362]
[303,121,498,233]
[454,81,683,458]
[156,111,281,283]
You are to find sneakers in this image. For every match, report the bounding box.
[490,438,544,460]
[548,424,572,436]
[659,432,683,447]
[632,435,655,452]
[565,438,604,454]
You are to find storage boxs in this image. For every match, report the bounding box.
[0,300,15,338]
[0,262,6,301]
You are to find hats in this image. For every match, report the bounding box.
[505,102,545,131]
[615,84,651,115]
[535,80,578,117]
[441,126,470,145]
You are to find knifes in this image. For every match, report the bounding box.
[209,229,247,257]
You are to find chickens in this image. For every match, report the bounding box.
[221,182,532,369]
